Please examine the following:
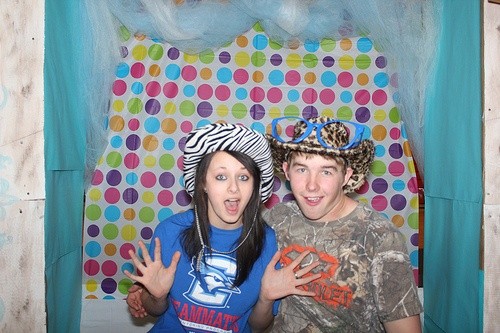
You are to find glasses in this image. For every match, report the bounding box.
[272,117,371,150]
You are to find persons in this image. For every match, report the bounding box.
[126,115,425,333]
[123,123,321,333]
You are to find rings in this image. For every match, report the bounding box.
[294,271,299,279]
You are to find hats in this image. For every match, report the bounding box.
[183,122,274,204]
[264,117,376,194]
